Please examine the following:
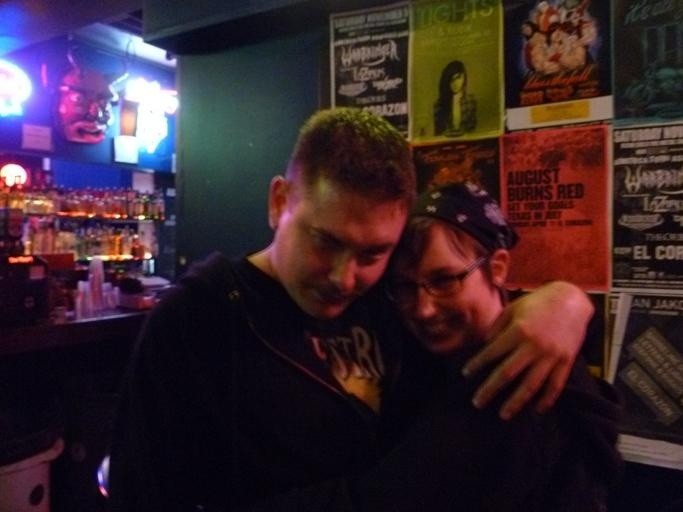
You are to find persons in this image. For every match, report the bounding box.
[380,179,627,511]
[431,61,478,138]
[50,44,131,146]
[506,1,612,109]
[104,108,595,511]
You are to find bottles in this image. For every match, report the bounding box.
[20,215,161,259]
[0,173,165,220]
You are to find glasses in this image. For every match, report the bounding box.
[383,254,489,303]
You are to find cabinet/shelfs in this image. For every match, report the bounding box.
[10,215,159,265]
